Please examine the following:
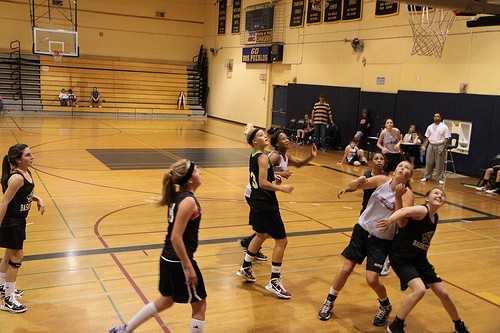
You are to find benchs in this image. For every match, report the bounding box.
[0,52,205,115]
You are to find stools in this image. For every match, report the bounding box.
[444,132,459,174]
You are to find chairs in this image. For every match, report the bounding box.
[477,157,494,186]
[283,119,340,145]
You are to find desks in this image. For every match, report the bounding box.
[368,137,421,168]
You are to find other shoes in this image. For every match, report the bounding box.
[107,324,127,333]
[437,179,443,184]
[492,190,500,195]
[486,189,496,194]
[421,177,429,181]
[475,186,485,190]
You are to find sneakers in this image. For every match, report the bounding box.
[0,294,27,313]
[254,251,267,261]
[265,275,291,298]
[373,304,391,326]
[318,293,335,320]
[1,286,24,297]
[387,323,405,333]
[454,325,470,333]
[241,240,247,254]
[236,265,256,281]
[380,260,391,275]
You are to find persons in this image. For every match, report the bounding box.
[177,90,186,110]
[0,144,44,313]
[237,93,500,333]
[68,89,79,107]
[58,88,67,106]
[0,96,3,115]
[90,87,102,108]
[107,159,208,333]
